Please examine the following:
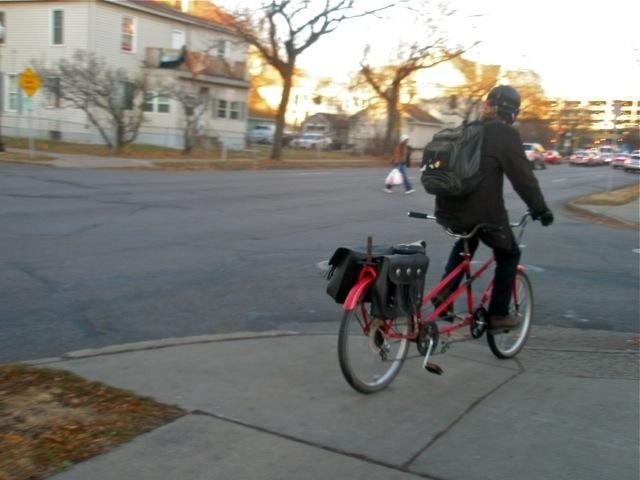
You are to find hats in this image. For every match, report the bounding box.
[399,135,408,142]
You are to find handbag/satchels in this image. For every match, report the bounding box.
[326,244,429,320]
[384,169,403,186]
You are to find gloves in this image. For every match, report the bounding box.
[539,210,554,226]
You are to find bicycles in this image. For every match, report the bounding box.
[338,210,535,394]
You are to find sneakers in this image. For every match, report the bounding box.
[432,288,454,322]
[490,315,521,332]
[383,188,415,194]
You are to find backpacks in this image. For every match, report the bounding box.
[421,121,498,197]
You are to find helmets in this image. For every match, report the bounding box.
[487,84,521,115]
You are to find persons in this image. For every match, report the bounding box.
[382,133,417,194]
[432,86,555,335]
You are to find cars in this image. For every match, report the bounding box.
[522,142,640,172]
[249,125,331,149]
